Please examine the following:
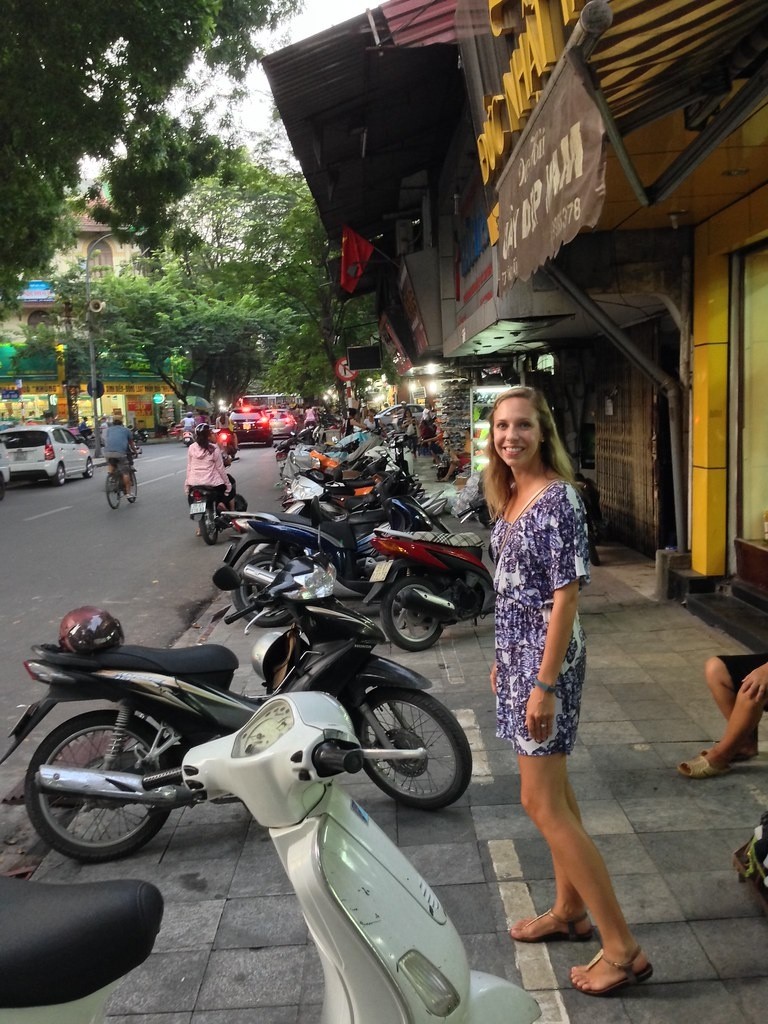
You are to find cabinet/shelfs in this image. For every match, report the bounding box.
[471,385,521,480]
[1,399,94,428]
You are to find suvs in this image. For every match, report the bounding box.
[227,406,274,446]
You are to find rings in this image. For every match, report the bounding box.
[541,724,547,727]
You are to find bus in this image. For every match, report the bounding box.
[234,395,326,416]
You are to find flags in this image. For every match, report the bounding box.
[339,225,374,293]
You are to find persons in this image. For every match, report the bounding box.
[304,405,316,426]
[99,418,107,448]
[437,427,470,483]
[81,417,95,439]
[180,412,197,432]
[197,411,211,425]
[486,388,653,994]
[216,410,239,451]
[185,423,238,536]
[346,410,369,437]
[677,654,768,778]
[420,406,444,465]
[363,408,381,431]
[401,409,417,458]
[101,415,140,498]
[400,401,413,417]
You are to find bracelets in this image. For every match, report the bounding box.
[535,679,556,693]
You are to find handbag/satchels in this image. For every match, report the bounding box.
[405,422,416,436]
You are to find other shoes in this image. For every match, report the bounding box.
[218,502,227,511]
[127,493,135,497]
[195,527,201,536]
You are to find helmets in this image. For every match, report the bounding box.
[207,434,217,444]
[186,412,193,418]
[195,423,209,434]
[58,604,125,653]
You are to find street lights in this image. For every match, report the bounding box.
[85,224,145,458]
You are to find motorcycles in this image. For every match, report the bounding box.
[0,496,474,864]
[215,418,497,652]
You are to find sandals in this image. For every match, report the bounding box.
[509,907,593,941]
[569,943,654,995]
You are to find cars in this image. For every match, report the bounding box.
[0,425,93,486]
[0,439,11,501]
[262,409,298,437]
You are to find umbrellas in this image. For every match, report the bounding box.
[178,396,213,410]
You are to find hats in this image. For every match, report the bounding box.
[113,415,123,421]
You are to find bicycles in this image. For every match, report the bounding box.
[103,448,143,509]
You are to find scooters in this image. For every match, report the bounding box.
[133,430,148,443]
[213,427,237,460]
[188,457,247,546]
[182,429,195,447]
[0,690,543,1024]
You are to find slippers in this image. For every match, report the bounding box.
[450,459,460,464]
[435,478,449,482]
[677,755,731,776]
[700,746,750,762]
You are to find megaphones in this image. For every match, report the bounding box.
[89,300,106,315]
